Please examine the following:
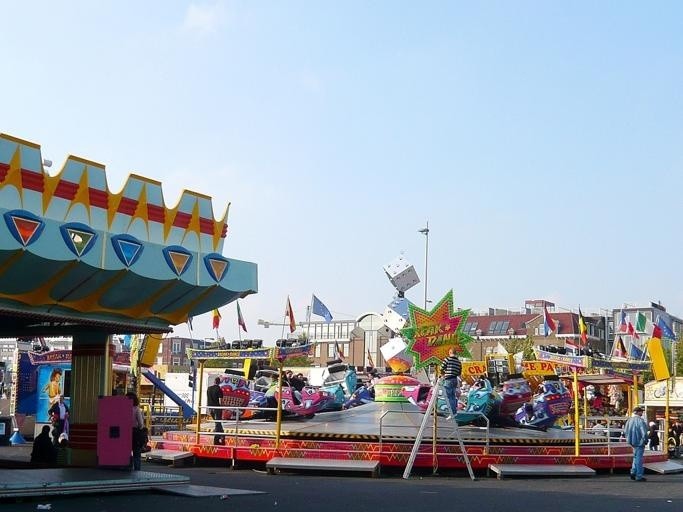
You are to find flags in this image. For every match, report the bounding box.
[619,307,638,339]
[236,302,248,331]
[186,315,199,332]
[630,347,651,368]
[541,308,555,340]
[577,312,589,343]
[632,310,662,338]
[654,315,678,342]
[311,295,332,322]
[652,323,663,339]
[367,350,374,368]
[338,344,345,361]
[565,338,580,348]
[611,337,624,357]
[285,298,295,332]
[334,343,338,357]
[208,305,220,329]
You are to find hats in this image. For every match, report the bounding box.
[633,407,645,412]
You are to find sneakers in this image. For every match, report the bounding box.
[214,438,225,445]
[630,474,647,482]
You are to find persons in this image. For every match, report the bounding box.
[366,372,381,392]
[30,424,57,465]
[364,381,374,400]
[291,372,305,405]
[582,419,682,452]
[207,375,225,445]
[281,369,302,402]
[624,408,649,482]
[45,396,68,441]
[123,391,147,471]
[45,368,62,407]
[440,347,462,421]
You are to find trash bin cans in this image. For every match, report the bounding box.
[0,416,13,446]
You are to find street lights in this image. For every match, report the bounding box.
[417,219,433,311]
